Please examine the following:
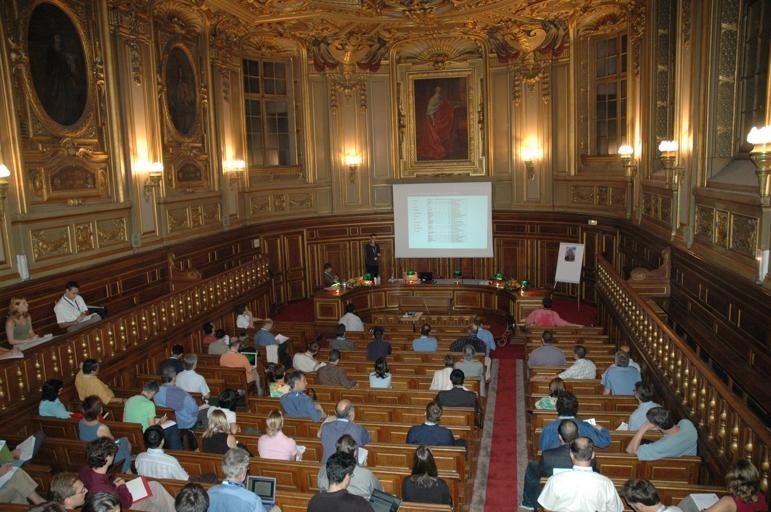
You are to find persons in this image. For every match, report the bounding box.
[238,333,266,387]
[401,445,453,506]
[202,409,254,457]
[369,357,392,388]
[80,491,120,512]
[292,342,327,372]
[203,321,217,345]
[135,424,217,484]
[601,345,640,378]
[123,380,182,449]
[316,350,360,389]
[358,447,368,466]
[0,439,24,466]
[316,399,372,464]
[534,378,567,411]
[253,318,290,360]
[328,324,356,350]
[470,317,496,356]
[530,346,596,381]
[0,462,48,506]
[699,459,768,512]
[527,331,567,368]
[39,380,82,420]
[434,369,484,430]
[152,365,208,430]
[219,336,264,395]
[306,451,375,512]
[364,233,381,278]
[159,344,185,375]
[268,363,292,398]
[412,324,438,352]
[323,263,337,287]
[621,477,683,511]
[367,326,392,362]
[76,436,176,512]
[208,329,230,355]
[78,395,133,475]
[236,303,264,329]
[207,389,242,435]
[258,408,301,461]
[26,501,67,512]
[405,401,468,462]
[524,297,584,328]
[0,346,17,355]
[429,354,478,398]
[454,344,486,399]
[625,407,699,462]
[628,381,663,432]
[449,323,491,384]
[337,304,365,332]
[537,436,624,512]
[54,281,89,329]
[175,483,209,512]
[317,434,384,503]
[521,419,597,510]
[603,351,642,396]
[175,353,211,400]
[207,448,282,512]
[75,358,124,405]
[50,472,88,512]
[539,392,611,452]
[5,295,40,345]
[278,372,327,423]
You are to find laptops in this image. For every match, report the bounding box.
[368,488,401,511]
[240,351,257,368]
[245,475,276,512]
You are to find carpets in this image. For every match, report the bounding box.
[483,345,524,511]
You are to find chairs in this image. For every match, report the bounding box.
[275,491,312,512]
[367,444,415,469]
[261,458,309,495]
[392,322,414,331]
[319,348,342,360]
[251,345,286,367]
[610,478,629,496]
[596,368,605,379]
[458,352,484,366]
[343,350,368,362]
[22,465,52,497]
[387,422,410,444]
[448,425,471,441]
[564,351,576,361]
[350,339,370,349]
[304,372,316,385]
[367,422,386,442]
[576,327,603,335]
[294,438,323,463]
[197,354,221,366]
[67,400,122,421]
[529,328,543,332]
[402,405,425,425]
[398,502,452,512]
[425,363,445,376]
[392,376,416,389]
[614,395,638,412]
[415,325,440,333]
[344,330,372,337]
[383,331,420,340]
[648,455,702,484]
[115,473,209,499]
[248,397,283,415]
[528,366,565,375]
[134,374,162,389]
[437,340,452,351]
[537,450,541,461]
[387,351,424,362]
[527,334,541,342]
[539,477,548,490]
[393,466,459,504]
[189,392,203,406]
[442,325,467,333]
[649,480,727,506]
[72,420,146,454]
[593,430,624,454]
[366,323,391,332]
[204,378,225,396]
[154,406,176,422]
[586,343,617,355]
[555,344,585,352]
[110,386,144,397]
[626,430,663,452]
[237,416,266,436]
[595,452,647,479]
[233,434,259,456]
[1,503,31,512]
[371,390,408,405]
[409,390,437,405]
[389,340,413,350]
[614,411,631,430]
[310,462,393,496]
[534,428,543,449]
[214,453,261,484]
[317,402,336,417]
[580,335,610,344]
[531,394,549,408]
[347,374,369,386]
[575,395,612,411]
[532,410,559,428]
[525,343,542,359]
[586,354,616,365]
[304,386,334,401]
[64,437,89,471]
[432,445,466,473]
[165,449,214,481]
[463,380,477,391]
[425,352,457,364]
[339,361,356,372]
[553,334,580,344]
[32,416,78,440]
[283,416,319,438]
[195,365,248,394]
[392,362,425,376]
[3,436,69,468]
[429,332,470,340]
[357,364,391,374]
[552,327,575,334]
[438,406,474,426]
[269,321,323,344]
[571,380,604,396]
[352,404,401,423]
[282,331,307,353]
[335,386,370,404]
[417,376,432,390]
[532,379,568,393]
[576,411,613,430]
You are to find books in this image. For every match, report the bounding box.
[125,475,152,505]
[296,445,306,461]
[0,466,19,488]
[275,333,289,344]
[155,417,177,429]
[616,422,629,431]
[583,418,596,426]
[80,312,97,323]
[224,334,229,345]
[15,435,36,462]
[678,493,719,512]
